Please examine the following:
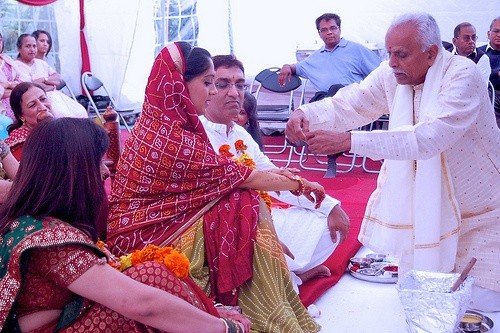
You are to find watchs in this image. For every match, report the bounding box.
[45,77,48,83]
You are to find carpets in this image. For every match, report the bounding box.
[101,127,384,310]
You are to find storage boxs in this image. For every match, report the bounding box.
[394,269,473,333]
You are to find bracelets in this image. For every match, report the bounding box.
[213,303,225,311]
[218,316,246,333]
[288,175,304,197]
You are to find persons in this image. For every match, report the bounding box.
[0,115,251,333]
[440,15,500,129]
[279,13,376,179]
[0,29,112,204]
[283,11,500,315]
[197,54,351,297]
[100,42,325,333]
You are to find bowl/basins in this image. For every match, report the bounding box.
[459,310,484,331]
[350,257,372,269]
[383,265,399,277]
[367,253,386,262]
[358,268,385,277]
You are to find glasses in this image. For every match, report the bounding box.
[213,80,249,94]
[455,34,478,42]
[318,26,339,35]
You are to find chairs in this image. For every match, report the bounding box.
[56,79,77,101]
[255,72,308,169]
[249,66,295,155]
[362,114,389,174]
[81,71,132,134]
[299,127,364,174]
[488,80,496,108]
